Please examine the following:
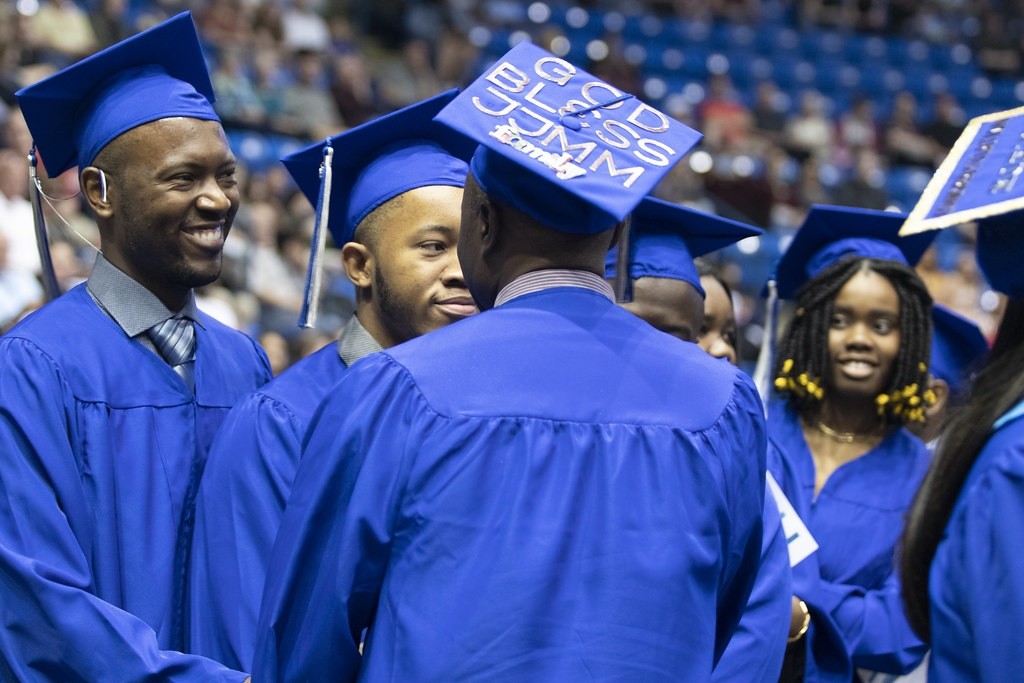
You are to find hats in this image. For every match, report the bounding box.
[431,41,703,239]
[14,9,221,301]
[279,83,480,329]
[601,196,765,297]
[751,202,941,422]
[899,106,1024,303]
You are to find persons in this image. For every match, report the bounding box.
[0,9,271,683]
[251,42,789,682]
[605,198,766,351]
[897,296,990,452]
[0,0,1024,334]
[184,85,482,683]
[691,267,741,365]
[896,106,1024,683]
[755,204,935,682]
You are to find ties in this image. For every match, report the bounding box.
[147,320,196,400]
